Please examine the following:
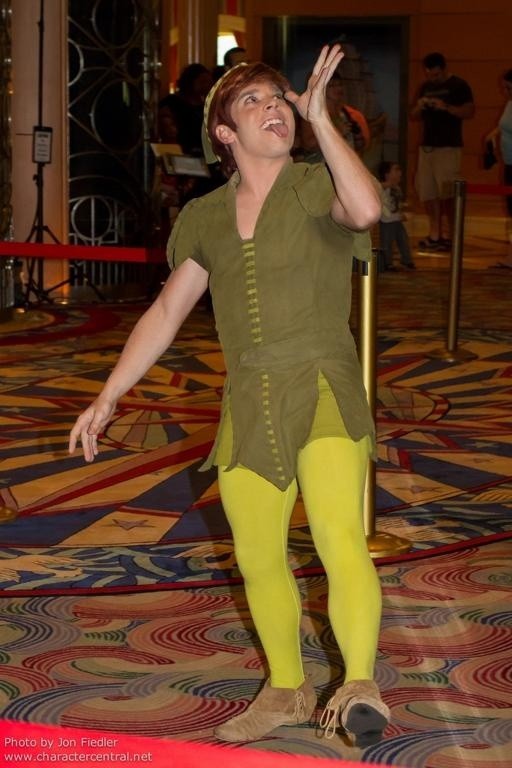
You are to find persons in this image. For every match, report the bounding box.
[410,51,476,250]
[67,43,391,748]
[224,46,248,71]
[302,70,388,169]
[376,157,414,270]
[177,63,230,196]
[485,70,512,269]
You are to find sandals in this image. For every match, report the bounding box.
[418,236,452,252]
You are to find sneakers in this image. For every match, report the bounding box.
[319,679,391,749]
[212,677,318,743]
